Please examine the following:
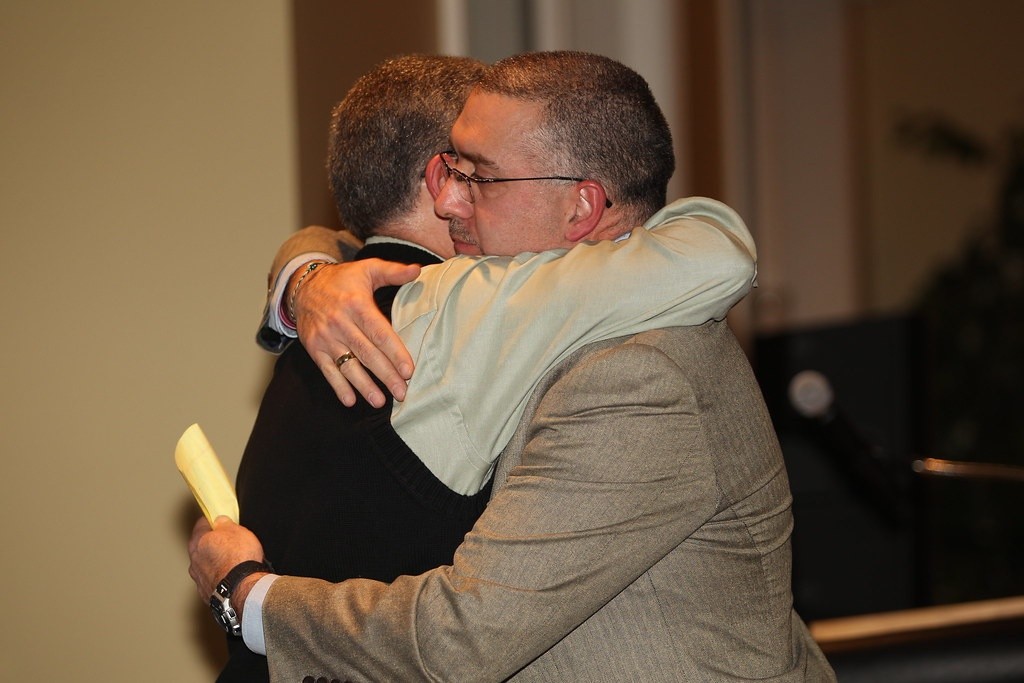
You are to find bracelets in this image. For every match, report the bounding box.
[289,262,339,323]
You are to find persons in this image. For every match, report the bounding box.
[218,53,759,683]
[185,53,837,683]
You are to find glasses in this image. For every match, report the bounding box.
[438,148,614,209]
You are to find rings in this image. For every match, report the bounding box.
[336,351,356,368]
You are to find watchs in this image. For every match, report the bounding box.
[208,561,275,643]
